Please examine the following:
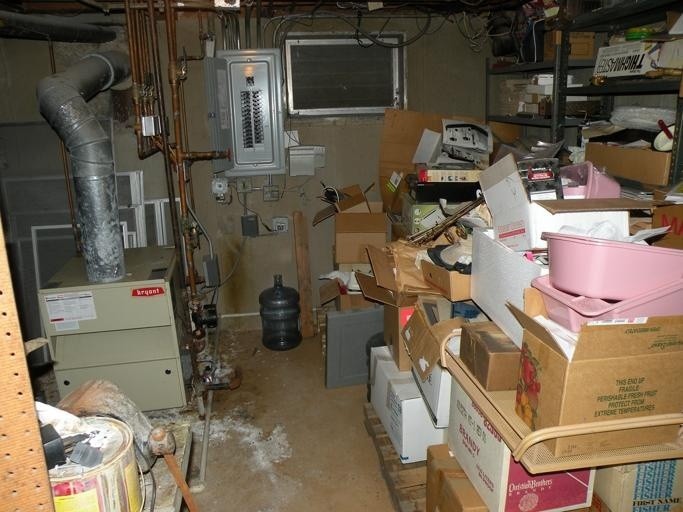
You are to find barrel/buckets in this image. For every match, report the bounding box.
[44,416,147,512]
[259,274,302,351]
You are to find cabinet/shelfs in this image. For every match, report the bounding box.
[556,0,682,194]
[483,52,614,167]
